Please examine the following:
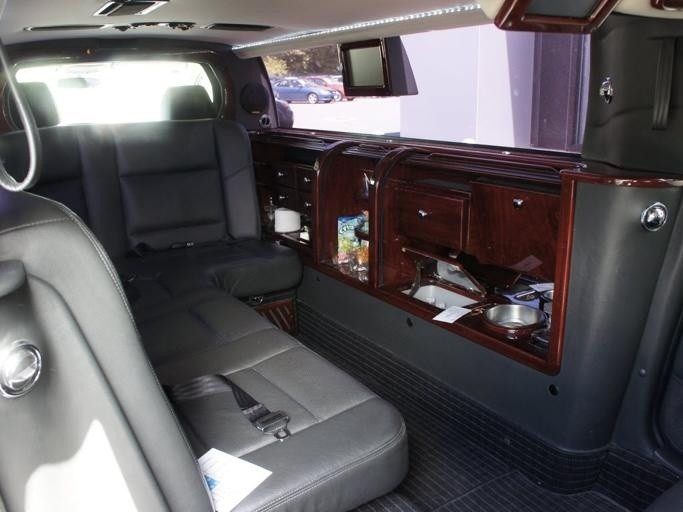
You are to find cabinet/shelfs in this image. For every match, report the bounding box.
[320,161,373,283]
[393,171,564,369]
[266,155,312,253]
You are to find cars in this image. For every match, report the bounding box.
[269,73,357,127]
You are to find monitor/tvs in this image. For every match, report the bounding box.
[339,36,393,96]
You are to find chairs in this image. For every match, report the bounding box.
[1,82,302,296]
[2,178,409,511]
